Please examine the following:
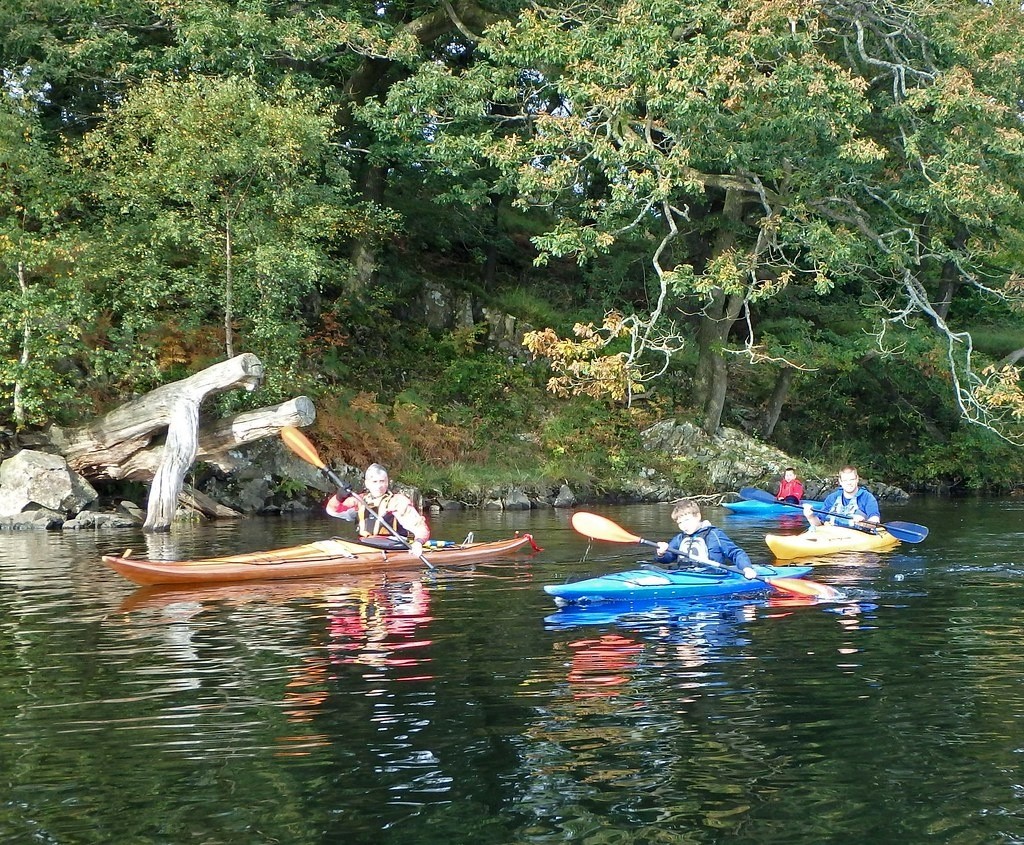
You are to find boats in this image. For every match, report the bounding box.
[765,526,902,560]
[101,534,533,585]
[542,564,816,603]
[721,499,825,514]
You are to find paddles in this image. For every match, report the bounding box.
[282,426,439,572]
[754,490,930,544]
[739,485,764,501]
[572,510,828,600]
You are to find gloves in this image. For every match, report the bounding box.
[336,482,353,503]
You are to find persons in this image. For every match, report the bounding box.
[325,464,431,557]
[656,501,757,580]
[803,466,881,528]
[776,468,803,505]
